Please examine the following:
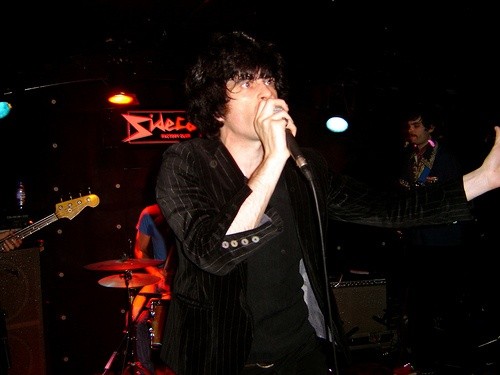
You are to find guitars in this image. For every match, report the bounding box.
[0,186,100,252]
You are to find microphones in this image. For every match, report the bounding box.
[286,128,314,182]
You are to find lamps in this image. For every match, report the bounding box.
[106,81,139,106]
[325,104,349,133]
[0,92,12,120]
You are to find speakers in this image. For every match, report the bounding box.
[330,279,390,346]
[0,247,47,375]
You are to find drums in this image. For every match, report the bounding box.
[150,299,171,350]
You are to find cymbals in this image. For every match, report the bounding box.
[98,273,161,287]
[83,258,165,271]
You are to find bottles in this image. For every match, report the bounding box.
[16,181,26,214]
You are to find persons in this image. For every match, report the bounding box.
[0,230,22,252]
[156,32,500,375]
[400,109,456,187]
[134,205,177,366]
